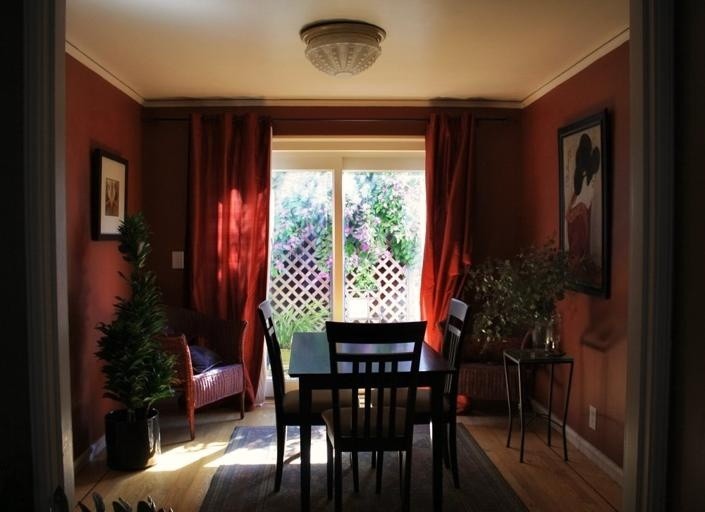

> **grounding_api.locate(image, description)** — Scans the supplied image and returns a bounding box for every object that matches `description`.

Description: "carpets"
[198,422,528,511]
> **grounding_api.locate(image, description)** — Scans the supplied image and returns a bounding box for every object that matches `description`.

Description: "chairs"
[257,299,360,501]
[458,333,531,413]
[322,320,427,510]
[371,298,472,496]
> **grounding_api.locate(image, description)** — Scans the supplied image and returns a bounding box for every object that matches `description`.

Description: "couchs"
[147,302,248,441]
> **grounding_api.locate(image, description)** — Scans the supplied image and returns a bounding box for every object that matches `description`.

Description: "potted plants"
[104,212,180,472]
[469,240,588,357]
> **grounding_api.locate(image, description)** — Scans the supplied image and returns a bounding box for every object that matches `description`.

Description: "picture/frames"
[558,108,612,300]
[91,146,129,241]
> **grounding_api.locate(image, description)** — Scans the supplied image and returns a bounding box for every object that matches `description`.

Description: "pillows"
[164,330,223,375]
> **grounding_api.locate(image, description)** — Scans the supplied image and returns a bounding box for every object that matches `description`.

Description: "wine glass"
[527,327,542,354]
[546,324,562,352]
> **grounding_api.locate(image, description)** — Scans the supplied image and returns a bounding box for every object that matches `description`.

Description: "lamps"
[300,21,387,78]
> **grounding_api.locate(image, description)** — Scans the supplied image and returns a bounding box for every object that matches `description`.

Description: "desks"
[501,347,574,464]
